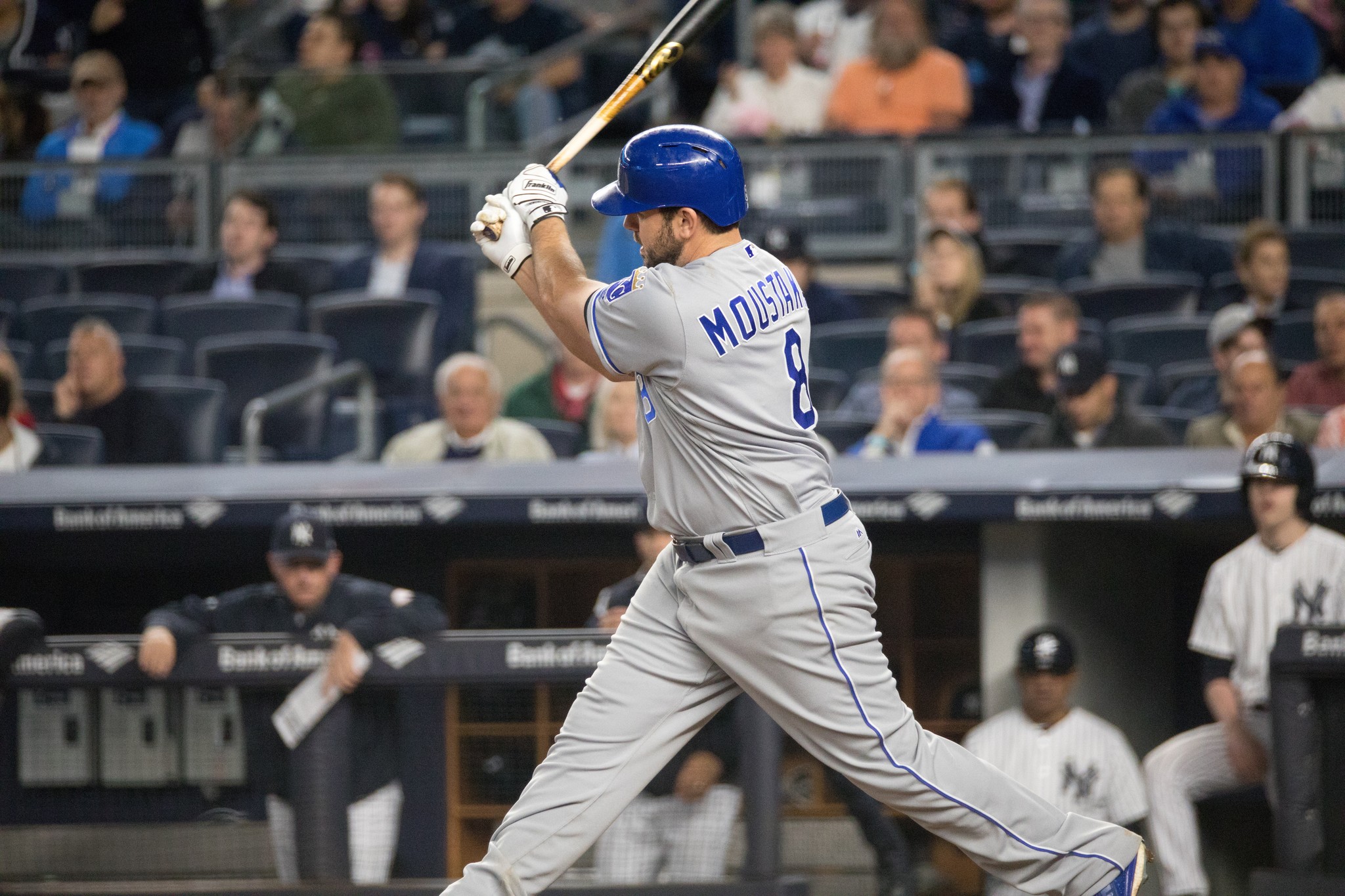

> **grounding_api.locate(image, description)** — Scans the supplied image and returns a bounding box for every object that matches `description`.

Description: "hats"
[74,50,124,95]
[1208,301,1272,352]
[269,511,335,564]
[1192,30,1236,63]
[1052,344,1108,396]
[1020,627,1077,677]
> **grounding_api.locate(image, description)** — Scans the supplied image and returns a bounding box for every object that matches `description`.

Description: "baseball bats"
[479,0,717,242]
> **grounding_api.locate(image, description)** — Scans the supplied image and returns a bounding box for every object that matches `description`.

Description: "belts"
[670,493,849,563]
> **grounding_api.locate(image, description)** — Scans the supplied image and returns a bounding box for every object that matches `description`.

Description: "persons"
[0,0,1345,896]
[434,124,1147,896]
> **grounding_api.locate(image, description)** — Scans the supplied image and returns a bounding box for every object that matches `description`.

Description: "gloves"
[507,162,568,230]
[471,193,533,278]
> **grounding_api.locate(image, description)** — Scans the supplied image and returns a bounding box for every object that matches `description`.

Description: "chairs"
[0,0,1345,462]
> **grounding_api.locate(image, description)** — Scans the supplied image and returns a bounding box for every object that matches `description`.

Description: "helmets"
[1237,432,1314,517]
[593,124,749,226]
